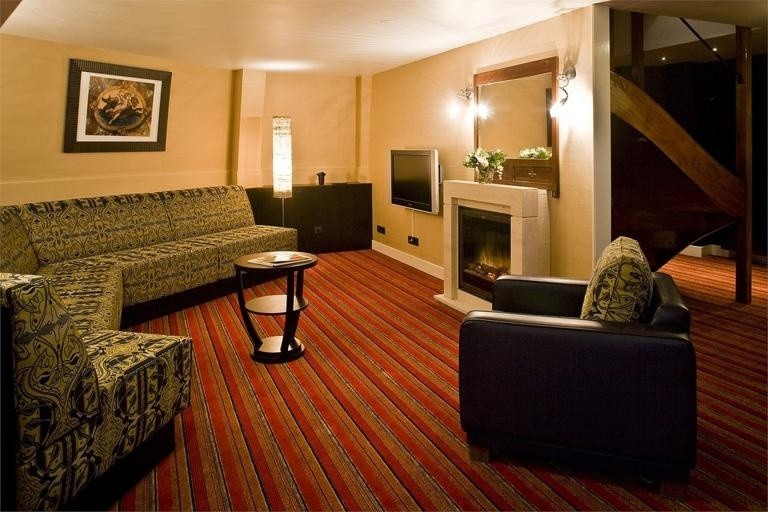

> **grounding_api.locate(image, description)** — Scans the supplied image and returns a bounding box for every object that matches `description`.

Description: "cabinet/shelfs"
[246,182,372,254]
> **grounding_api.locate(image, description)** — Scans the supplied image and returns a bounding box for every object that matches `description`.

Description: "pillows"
[580,235,654,322]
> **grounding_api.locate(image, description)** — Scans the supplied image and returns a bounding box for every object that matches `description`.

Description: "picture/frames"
[63,59,172,153]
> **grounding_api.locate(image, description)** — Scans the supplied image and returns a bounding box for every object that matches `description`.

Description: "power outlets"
[408,235,419,246]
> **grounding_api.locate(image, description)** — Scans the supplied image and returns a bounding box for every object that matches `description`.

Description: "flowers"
[518,146,552,159]
[462,147,508,181]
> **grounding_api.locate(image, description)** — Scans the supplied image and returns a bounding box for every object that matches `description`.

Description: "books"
[248,253,312,267]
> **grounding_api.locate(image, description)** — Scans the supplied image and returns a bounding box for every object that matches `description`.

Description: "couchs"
[459,271,697,492]
[0,185,298,511]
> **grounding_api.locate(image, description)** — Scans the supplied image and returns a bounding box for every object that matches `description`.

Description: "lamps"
[456,85,476,102]
[272,116,293,227]
[550,65,576,118]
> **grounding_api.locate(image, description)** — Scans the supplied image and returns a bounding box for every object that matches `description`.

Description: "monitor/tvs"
[390,149,442,215]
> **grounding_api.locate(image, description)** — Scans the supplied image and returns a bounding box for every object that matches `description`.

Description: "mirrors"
[472,56,559,198]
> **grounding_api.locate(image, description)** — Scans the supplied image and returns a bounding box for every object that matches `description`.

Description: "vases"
[478,169,494,183]
